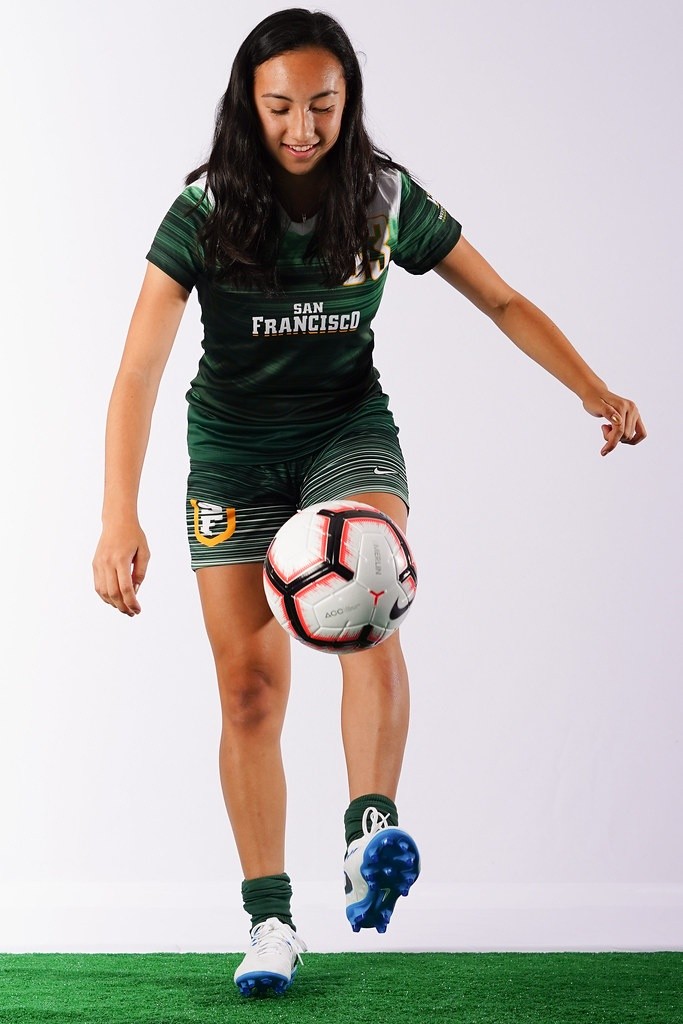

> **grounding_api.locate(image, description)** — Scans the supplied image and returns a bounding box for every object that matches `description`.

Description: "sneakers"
[234,918,307,997]
[340,807,421,935]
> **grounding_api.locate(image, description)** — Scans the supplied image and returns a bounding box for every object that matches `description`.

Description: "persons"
[93,8,647,995]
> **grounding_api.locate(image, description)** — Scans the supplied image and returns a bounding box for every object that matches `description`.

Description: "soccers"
[262,498,420,655]
